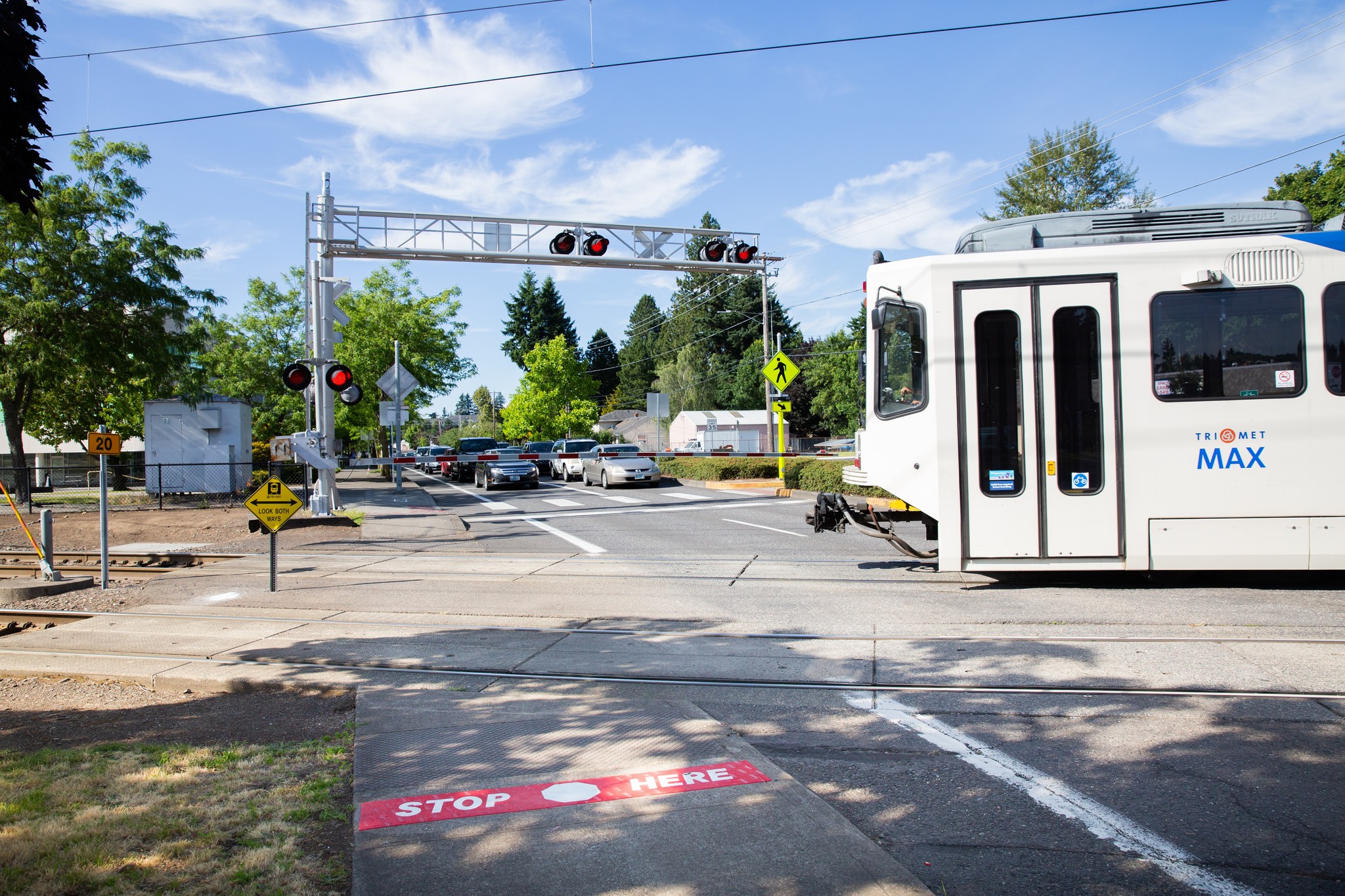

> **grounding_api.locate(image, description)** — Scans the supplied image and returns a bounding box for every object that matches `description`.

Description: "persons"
[900,387,923,405]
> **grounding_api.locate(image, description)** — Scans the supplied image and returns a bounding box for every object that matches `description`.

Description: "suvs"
[550,438,600,482]
[413,446,427,469]
[496,442,511,449]
[450,437,499,483]
[424,446,453,474]
[521,440,555,473]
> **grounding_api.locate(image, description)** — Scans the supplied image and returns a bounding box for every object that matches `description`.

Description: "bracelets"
[914,389,916,395]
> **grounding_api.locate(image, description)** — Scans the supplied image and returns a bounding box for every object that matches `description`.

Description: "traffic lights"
[283,363,354,391]
[706,241,758,264]
[553,232,610,256]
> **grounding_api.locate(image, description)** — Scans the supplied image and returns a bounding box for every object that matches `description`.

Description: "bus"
[799,198,1345,583]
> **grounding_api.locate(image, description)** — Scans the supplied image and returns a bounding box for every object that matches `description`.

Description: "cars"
[392,452,416,458]
[507,446,525,451]
[440,448,455,477]
[420,447,429,471]
[473,448,540,489]
[582,443,661,490]
